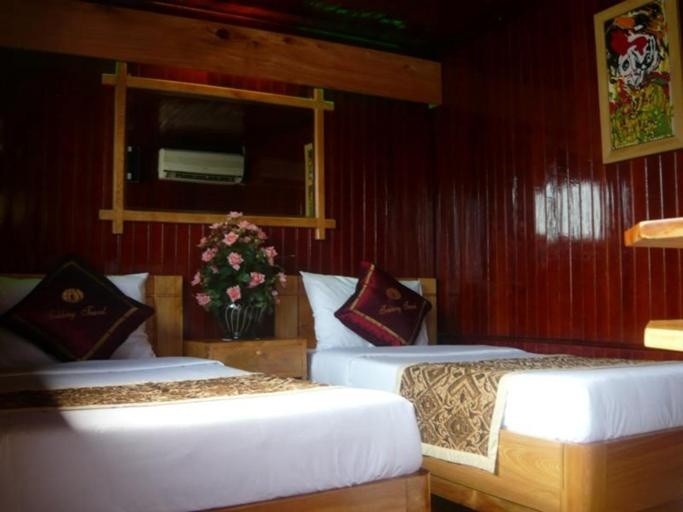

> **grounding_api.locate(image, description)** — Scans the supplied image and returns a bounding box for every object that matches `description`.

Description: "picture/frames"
[593,0,683,166]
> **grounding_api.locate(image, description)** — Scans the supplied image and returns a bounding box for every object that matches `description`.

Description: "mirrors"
[95,60,337,243]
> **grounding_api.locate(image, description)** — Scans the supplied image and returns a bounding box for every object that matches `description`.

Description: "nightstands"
[184,335,308,382]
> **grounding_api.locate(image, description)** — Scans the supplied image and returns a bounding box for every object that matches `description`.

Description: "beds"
[0,267,433,512]
[271,273,683,512]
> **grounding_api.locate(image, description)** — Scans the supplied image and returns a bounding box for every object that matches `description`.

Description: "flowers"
[189,208,288,310]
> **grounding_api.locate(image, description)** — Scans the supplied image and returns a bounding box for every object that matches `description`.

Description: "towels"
[0,372,330,416]
[399,353,667,475]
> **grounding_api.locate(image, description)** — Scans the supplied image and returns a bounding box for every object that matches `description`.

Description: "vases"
[215,297,263,340]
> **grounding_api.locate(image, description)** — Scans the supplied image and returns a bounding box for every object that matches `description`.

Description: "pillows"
[297,269,430,351]
[0,250,158,364]
[0,271,156,361]
[333,262,434,346]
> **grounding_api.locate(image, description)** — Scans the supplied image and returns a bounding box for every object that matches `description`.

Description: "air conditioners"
[157,148,246,186]
